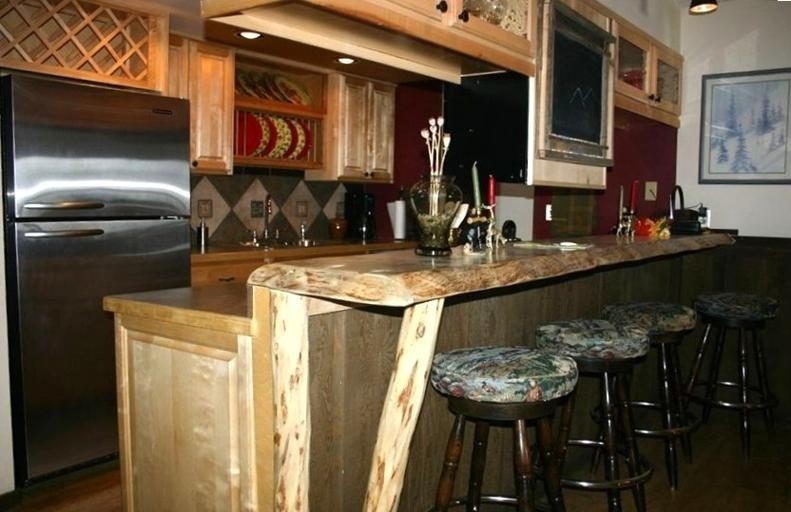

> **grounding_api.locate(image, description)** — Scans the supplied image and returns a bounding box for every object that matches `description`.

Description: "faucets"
[262,192,273,240]
[298,223,307,239]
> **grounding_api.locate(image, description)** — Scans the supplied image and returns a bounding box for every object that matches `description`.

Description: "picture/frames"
[696,68,791,185]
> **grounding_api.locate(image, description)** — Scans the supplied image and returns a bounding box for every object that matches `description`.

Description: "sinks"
[239,237,323,248]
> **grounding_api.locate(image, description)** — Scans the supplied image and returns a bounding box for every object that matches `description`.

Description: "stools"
[534,319,651,511]
[600,299,699,492]
[684,293,780,460]
[427,345,581,512]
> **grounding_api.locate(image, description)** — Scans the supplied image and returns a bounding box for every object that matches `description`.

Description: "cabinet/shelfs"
[0,1,171,98]
[303,71,398,184]
[233,48,328,170]
[616,15,684,118]
[190,255,267,286]
[201,0,539,87]
[168,30,234,176]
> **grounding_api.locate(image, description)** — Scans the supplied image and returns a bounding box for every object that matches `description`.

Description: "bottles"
[197,215,210,250]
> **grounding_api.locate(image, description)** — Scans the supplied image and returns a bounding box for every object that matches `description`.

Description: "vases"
[404,167,464,259]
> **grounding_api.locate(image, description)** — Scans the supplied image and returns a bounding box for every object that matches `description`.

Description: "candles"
[618,183,624,223]
[470,159,483,217]
[487,173,496,216]
[629,180,640,215]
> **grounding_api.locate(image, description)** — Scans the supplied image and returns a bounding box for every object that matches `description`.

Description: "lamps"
[686,0,718,15]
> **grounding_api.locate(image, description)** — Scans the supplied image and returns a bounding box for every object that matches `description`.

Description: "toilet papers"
[386,199,407,241]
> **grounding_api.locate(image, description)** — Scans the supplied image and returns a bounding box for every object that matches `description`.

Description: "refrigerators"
[0,71,193,491]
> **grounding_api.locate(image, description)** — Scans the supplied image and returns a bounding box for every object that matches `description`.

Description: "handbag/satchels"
[663,209,701,235]
